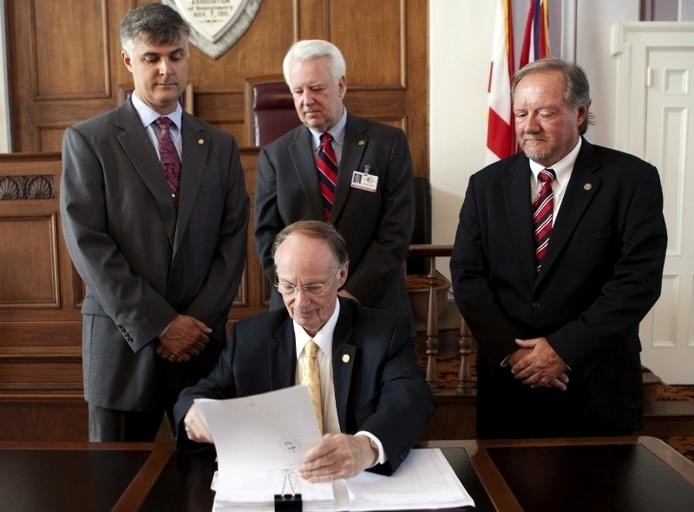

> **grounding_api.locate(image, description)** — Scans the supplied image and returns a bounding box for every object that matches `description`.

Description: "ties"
[303,341,323,436]
[315,133,339,225]
[154,117,181,207]
[531,168,556,273]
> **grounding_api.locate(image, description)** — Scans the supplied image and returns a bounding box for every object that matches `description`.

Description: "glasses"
[274,265,342,297]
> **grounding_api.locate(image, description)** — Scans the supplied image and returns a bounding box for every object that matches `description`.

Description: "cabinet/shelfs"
[8,2,428,151]
[0,155,263,391]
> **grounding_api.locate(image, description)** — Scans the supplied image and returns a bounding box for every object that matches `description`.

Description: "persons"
[449,58,668,438]
[60,4,250,445]
[171,220,436,486]
[253,39,415,338]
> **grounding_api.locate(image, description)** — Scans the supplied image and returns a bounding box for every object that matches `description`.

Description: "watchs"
[364,433,380,468]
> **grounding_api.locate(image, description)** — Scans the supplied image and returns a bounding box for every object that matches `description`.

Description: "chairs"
[238,71,313,149]
[401,179,452,322]
[115,82,194,127]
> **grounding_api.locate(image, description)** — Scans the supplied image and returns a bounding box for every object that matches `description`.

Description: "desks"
[3,436,694,511]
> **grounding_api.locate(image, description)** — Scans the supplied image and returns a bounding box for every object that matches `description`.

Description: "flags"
[518,0,552,72]
[486,0,514,161]
